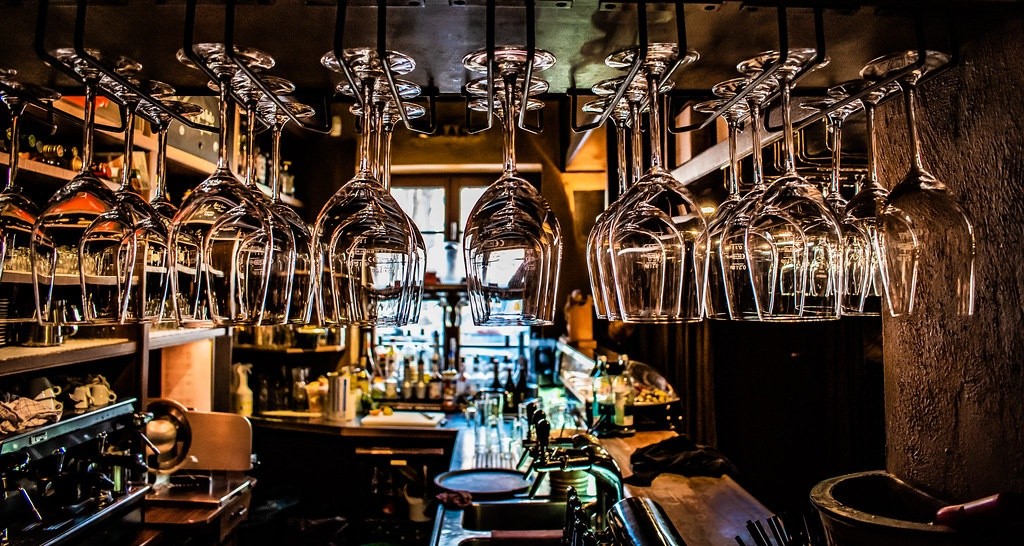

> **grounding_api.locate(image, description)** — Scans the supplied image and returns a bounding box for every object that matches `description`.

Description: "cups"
[92,385,117,405]
[32,377,61,399]
[473,392,524,460]
[74,386,94,409]
[39,397,64,423]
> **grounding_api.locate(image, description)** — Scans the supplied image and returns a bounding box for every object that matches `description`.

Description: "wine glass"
[0,41,976,330]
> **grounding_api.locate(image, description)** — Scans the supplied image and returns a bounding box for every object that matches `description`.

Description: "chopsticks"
[735,506,821,546]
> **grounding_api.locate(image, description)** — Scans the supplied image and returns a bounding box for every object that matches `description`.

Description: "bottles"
[275,365,291,408]
[593,356,614,438]
[293,369,307,407]
[359,353,531,412]
[612,354,636,434]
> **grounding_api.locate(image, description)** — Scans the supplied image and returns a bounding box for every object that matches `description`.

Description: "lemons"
[369,407,393,415]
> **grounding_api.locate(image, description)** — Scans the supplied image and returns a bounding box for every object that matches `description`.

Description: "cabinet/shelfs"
[0,314,149,546]
[0,96,306,376]
